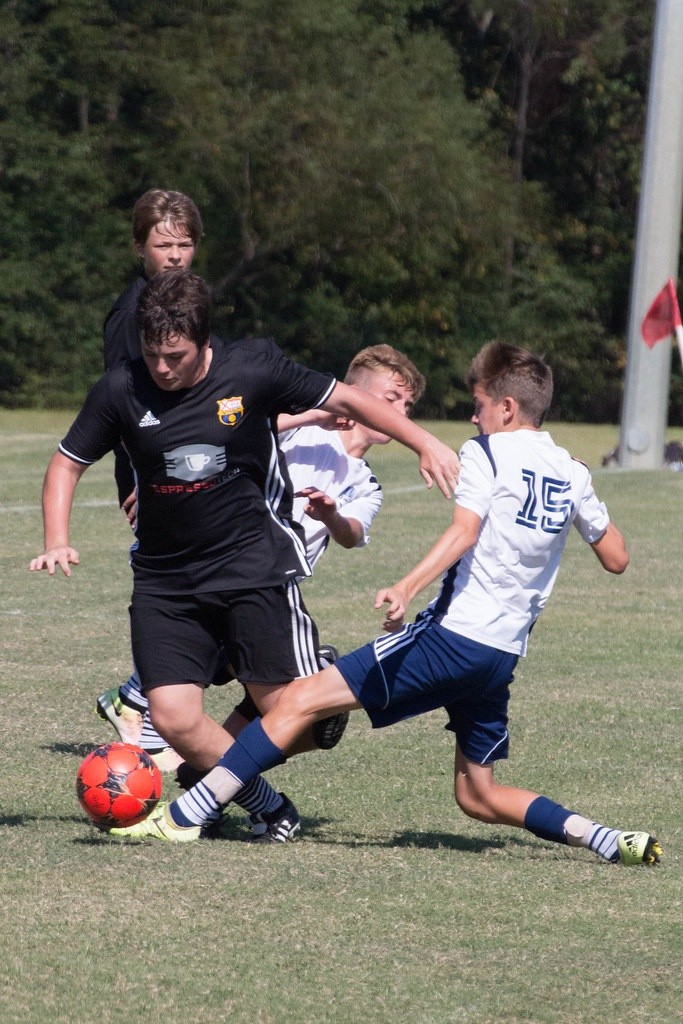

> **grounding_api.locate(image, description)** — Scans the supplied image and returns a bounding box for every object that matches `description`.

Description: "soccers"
[77,741,162,829]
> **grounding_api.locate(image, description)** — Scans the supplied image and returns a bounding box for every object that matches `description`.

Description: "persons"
[174,345,429,836]
[110,342,662,869]
[31,269,459,843]
[95,189,229,757]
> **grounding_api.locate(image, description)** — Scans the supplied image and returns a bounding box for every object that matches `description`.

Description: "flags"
[641,277,681,351]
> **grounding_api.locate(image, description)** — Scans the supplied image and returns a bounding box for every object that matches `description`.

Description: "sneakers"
[109,802,202,843]
[93,686,164,755]
[245,792,301,843]
[617,832,662,866]
[311,644,349,750]
